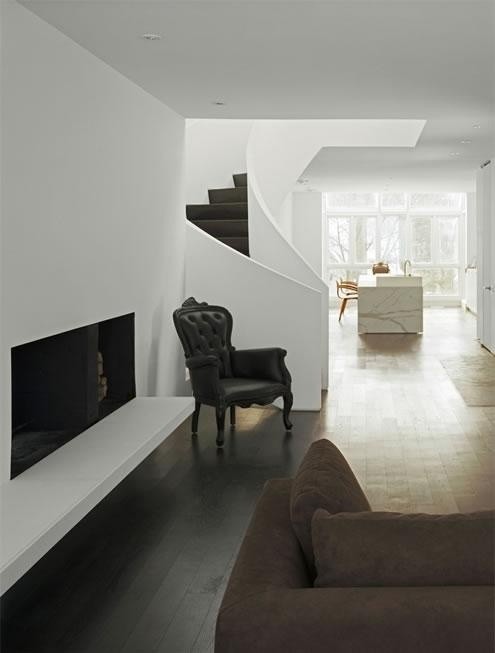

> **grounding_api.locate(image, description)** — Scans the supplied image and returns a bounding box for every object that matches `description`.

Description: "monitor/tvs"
[11,311,136,481]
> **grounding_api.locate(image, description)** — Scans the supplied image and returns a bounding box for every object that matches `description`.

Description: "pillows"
[288,438,373,576]
[305,504,494,587]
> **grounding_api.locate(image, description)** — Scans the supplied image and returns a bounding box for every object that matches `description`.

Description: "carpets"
[440,345,495,408]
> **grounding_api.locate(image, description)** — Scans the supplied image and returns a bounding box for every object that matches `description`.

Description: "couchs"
[211,437,494,651]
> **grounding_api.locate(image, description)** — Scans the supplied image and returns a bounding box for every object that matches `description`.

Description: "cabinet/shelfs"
[475,155,495,356]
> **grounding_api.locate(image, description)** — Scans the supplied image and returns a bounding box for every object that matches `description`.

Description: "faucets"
[404,259,411,276]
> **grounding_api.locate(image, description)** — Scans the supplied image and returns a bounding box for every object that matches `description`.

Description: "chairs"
[171,294,297,448]
[334,280,358,319]
[340,276,358,311]
